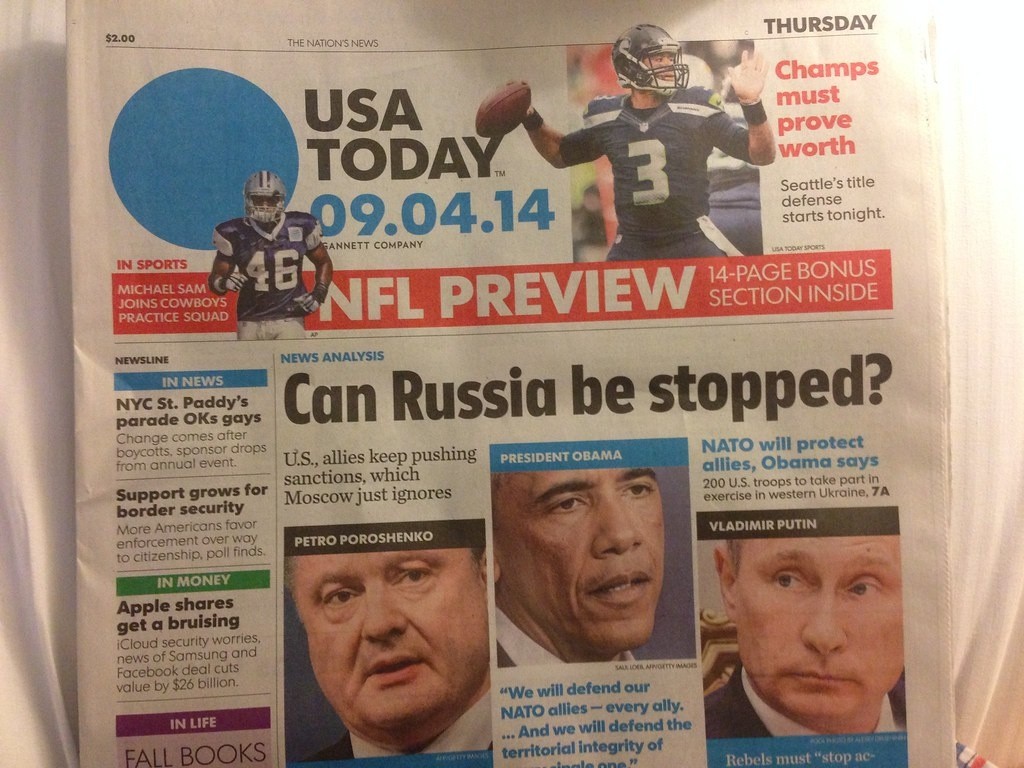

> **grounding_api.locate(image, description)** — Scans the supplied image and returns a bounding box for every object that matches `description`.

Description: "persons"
[504,24,779,262]
[285,547,492,768]
[208,173,334,340]
[705,536,906,738]
[491,468,665,666]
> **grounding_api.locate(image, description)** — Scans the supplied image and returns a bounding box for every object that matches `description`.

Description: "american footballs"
[475,82,531,138]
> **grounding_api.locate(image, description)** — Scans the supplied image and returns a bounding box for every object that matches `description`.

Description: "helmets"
[243,171,285,223]
[612,24,689,97]
[676,54,715,90]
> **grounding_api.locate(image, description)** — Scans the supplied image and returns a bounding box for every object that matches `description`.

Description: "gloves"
[216,274,248,293]
[293,283,328,316]
